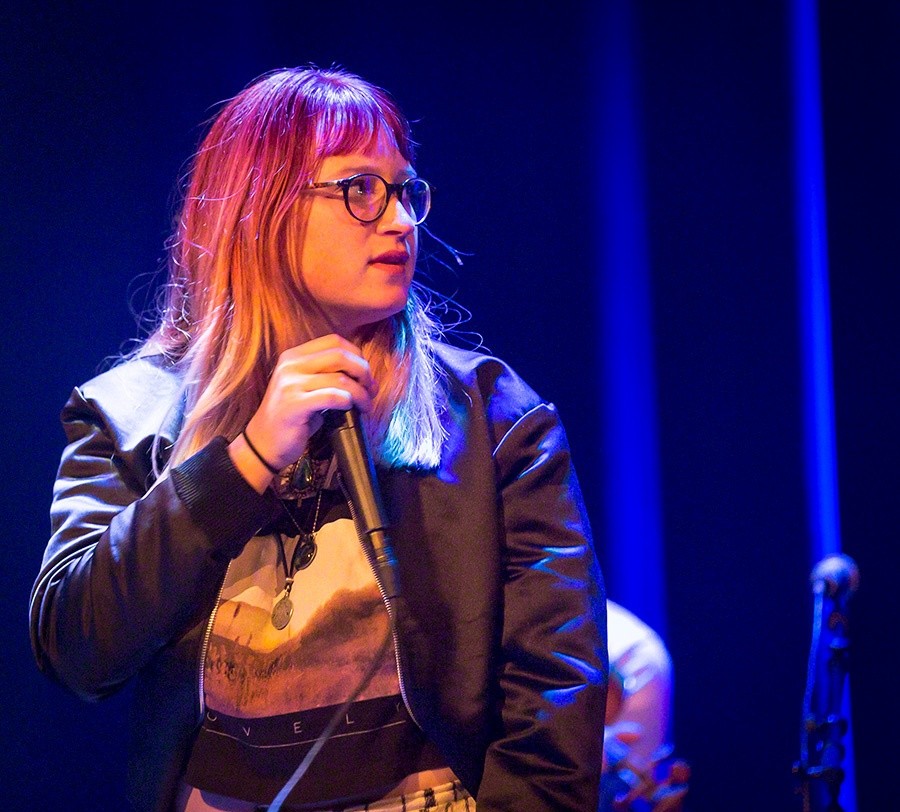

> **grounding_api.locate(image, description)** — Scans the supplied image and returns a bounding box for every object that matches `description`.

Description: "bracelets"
[242,424,283,481]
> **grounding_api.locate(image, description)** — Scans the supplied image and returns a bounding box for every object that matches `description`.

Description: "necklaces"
[260,426,336,630]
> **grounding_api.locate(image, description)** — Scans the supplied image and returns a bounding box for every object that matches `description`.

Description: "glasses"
[301,172,431,226]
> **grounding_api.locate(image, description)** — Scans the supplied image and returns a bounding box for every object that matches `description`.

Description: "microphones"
[315,404,403,599]
[811,554,861,597]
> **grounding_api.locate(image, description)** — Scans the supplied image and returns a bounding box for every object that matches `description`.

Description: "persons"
[596,602,683,812]
[26,65,610,812]
